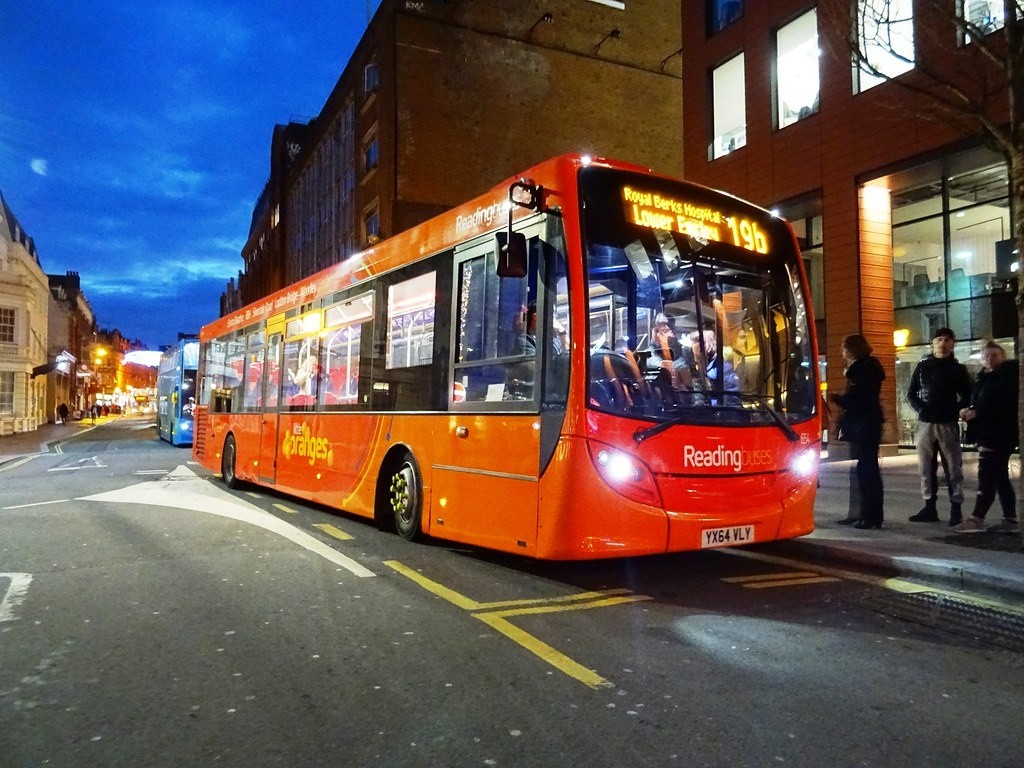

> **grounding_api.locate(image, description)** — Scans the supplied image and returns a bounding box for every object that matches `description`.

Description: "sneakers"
[987,518,1021,533]
[949,517,986,532]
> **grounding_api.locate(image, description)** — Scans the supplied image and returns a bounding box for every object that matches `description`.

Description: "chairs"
[216,357,359,406]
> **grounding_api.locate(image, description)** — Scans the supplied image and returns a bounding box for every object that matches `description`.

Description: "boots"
[950,503,962,526]
[908,499,939,522]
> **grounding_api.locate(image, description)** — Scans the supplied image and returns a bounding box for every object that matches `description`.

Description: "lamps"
[528,13,555,32]
[595,28,620,55]
[661,47,688,71]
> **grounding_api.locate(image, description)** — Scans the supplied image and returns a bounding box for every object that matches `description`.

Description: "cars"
[124,403,157,418]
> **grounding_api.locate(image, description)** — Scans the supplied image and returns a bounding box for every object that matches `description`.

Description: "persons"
[90,404,110,424]
[828,334,886,529]
[641,313,742,409]
[510,299,570,398]
[223,364,358,406]
[907,328,975,526]
[182,397,196,418]
[948,341,1021,533]
[59,403,68,424]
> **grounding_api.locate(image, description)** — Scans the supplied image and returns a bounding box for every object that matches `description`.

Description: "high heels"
[839,518,857,524]
[853,517,882,529]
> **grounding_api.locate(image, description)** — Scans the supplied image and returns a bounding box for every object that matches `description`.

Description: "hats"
[934,327,955,340]
[653,313,675,331]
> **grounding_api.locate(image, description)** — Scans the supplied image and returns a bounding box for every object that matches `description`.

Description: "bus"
[156,338,264,446]
[192,152,825,564]
